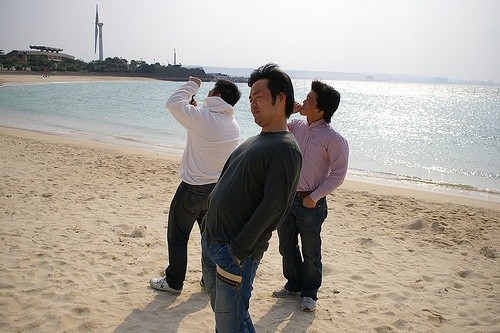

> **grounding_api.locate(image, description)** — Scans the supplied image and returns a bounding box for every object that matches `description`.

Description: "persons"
[200,63,303,333]
[272,80,349,312]
[149,76,242,295]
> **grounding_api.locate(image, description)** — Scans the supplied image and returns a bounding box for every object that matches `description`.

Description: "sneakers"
[149,276,182,293]
[272,286,301,297]
[300,296,316,311]
